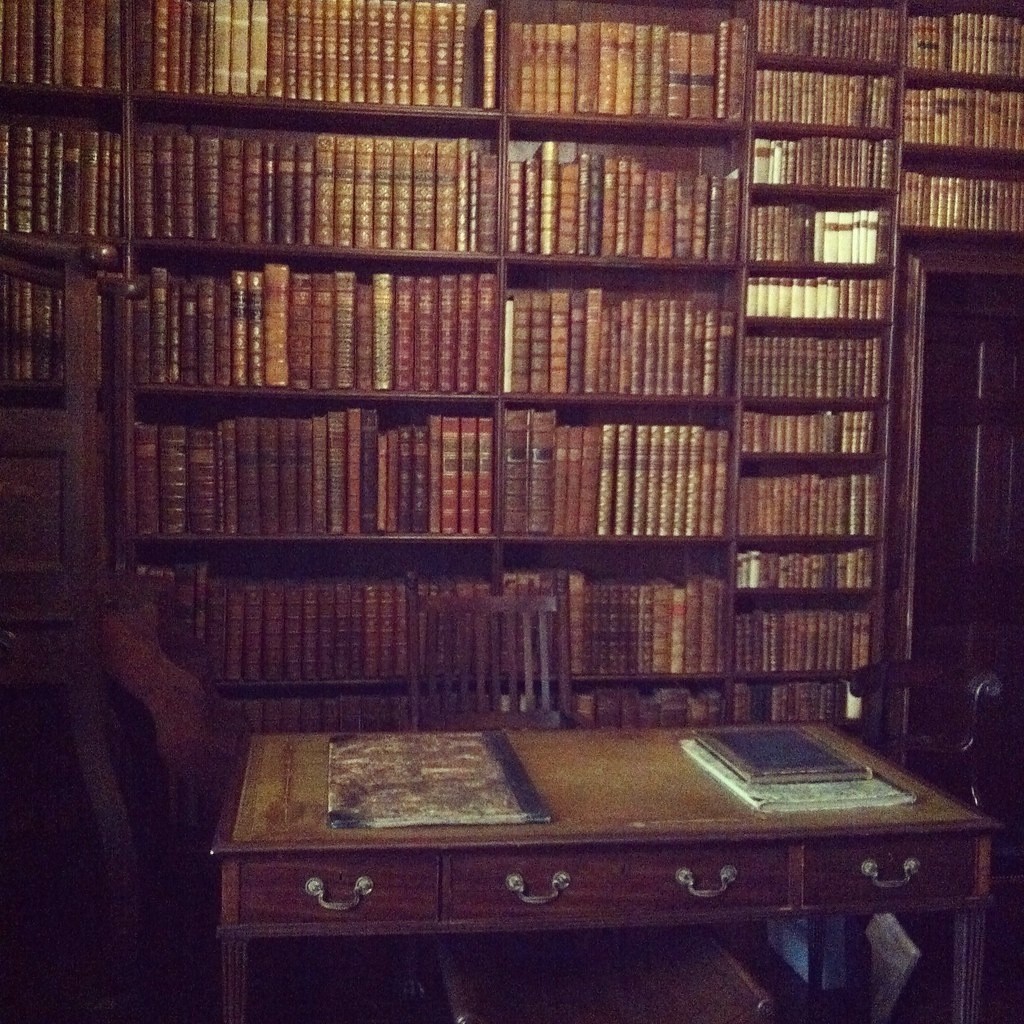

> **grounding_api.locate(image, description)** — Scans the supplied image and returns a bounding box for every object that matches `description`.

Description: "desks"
[211,721,1005,1024]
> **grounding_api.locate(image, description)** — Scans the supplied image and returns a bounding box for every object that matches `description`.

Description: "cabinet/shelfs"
[0,0,1022,855]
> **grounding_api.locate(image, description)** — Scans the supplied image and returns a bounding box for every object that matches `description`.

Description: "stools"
[443,925,779,1024]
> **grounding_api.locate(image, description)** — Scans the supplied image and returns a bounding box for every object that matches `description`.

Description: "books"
[324,733,546,830]
[696,727,874,787]
[1,0,1023,731]
[679,731,921,816]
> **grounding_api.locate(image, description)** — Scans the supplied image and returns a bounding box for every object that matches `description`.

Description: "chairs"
[399,565,570,743]
[804,662,1004,1024]
[75,557,407,1023]
